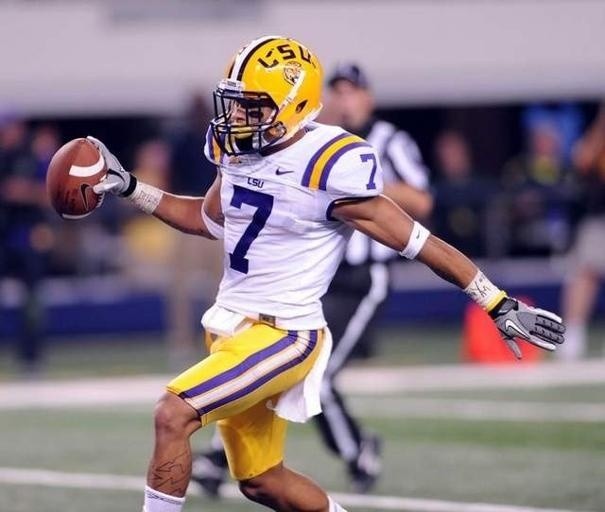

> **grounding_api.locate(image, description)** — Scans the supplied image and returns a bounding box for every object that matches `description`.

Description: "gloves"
[487,295,566,359]
[86,135,137,198]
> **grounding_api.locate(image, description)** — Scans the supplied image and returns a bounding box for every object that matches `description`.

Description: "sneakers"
[190,452,229,494]
[349,437,383,494]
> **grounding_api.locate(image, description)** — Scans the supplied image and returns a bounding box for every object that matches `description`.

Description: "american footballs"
[47,138,108,222]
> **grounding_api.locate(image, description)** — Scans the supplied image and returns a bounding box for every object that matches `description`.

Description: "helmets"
[210,35,323,157]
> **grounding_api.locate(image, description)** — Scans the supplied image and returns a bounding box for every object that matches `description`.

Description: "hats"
[327,65,366,89]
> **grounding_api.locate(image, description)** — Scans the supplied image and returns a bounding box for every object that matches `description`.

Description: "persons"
[423,96,605,362]
[2,91,224,376]
[84,34,566,512]
[189,59,436,502]
